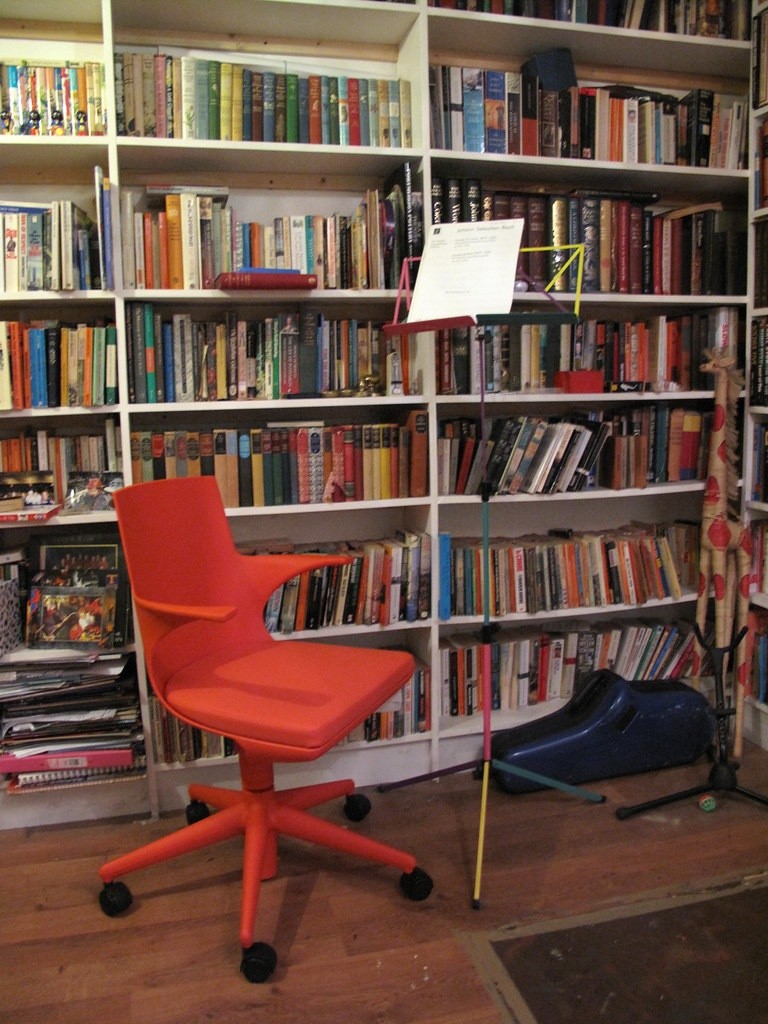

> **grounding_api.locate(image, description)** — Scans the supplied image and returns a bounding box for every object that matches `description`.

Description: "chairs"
[98,476,437,984]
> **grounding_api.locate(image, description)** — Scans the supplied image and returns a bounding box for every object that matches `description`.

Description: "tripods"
[378,246,607,913]
[617,624,768,821]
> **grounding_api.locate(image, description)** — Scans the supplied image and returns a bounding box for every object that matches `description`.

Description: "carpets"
[456,866,768,1024]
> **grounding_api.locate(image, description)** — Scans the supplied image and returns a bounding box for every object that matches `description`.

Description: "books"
[0,0,768,797]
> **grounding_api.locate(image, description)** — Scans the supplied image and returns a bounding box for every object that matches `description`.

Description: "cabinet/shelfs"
[729,0,768,750]
[0,0,751,832]
